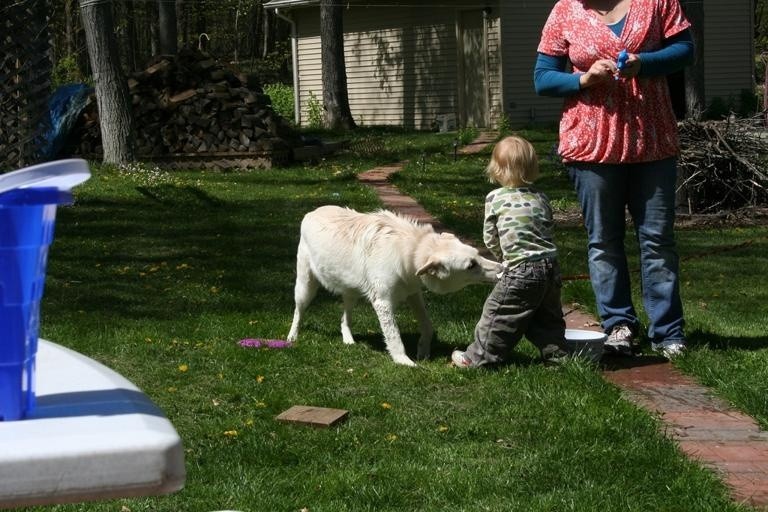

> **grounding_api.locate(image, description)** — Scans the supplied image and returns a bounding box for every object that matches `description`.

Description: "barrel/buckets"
[0,185,76,421]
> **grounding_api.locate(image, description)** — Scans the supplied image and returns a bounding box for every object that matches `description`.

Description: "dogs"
[286,204,504,368]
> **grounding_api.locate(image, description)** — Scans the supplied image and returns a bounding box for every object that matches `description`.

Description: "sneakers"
[451,349,472,369]
[542,343,569,371]
[656,344,689,363]
[602,323,633,357]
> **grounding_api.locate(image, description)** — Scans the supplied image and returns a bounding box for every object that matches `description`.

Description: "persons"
[533,0,701,364]
[450,136,571,369]
